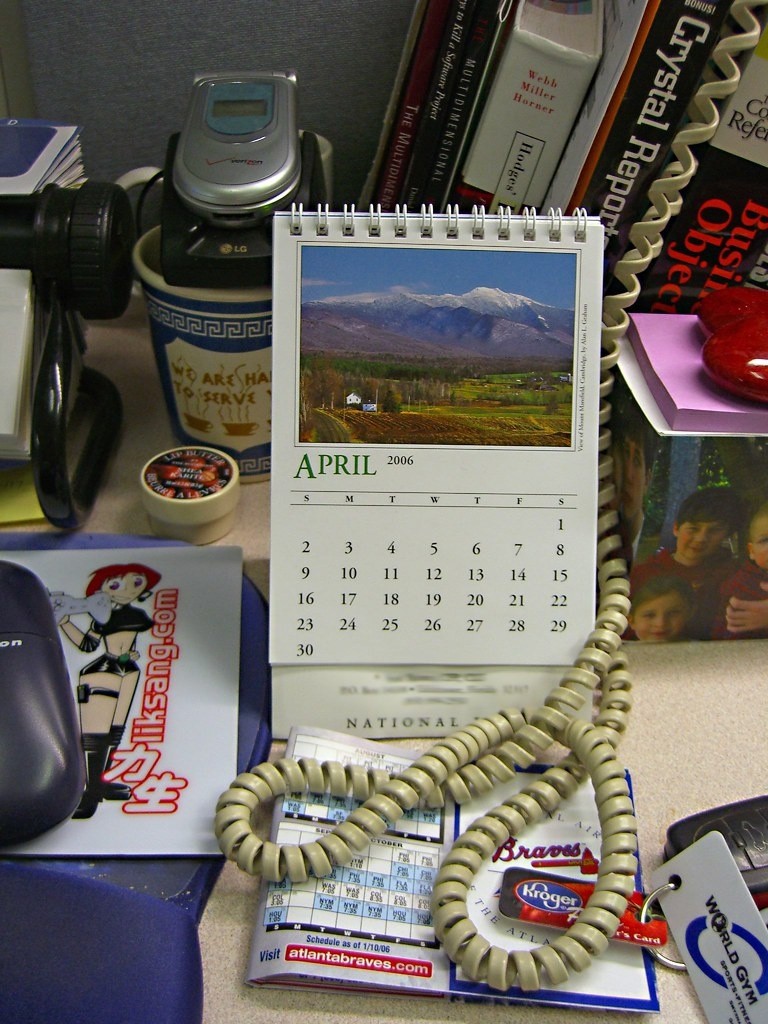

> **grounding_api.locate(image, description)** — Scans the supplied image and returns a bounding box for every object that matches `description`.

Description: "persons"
[615,398,768,643]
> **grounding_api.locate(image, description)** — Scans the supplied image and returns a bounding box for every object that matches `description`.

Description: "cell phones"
[173,69,301,228]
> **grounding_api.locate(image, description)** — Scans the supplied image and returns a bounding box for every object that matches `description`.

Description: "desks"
[0,276,768,1024]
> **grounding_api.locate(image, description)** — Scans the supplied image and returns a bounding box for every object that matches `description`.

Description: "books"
[375,2,768,321]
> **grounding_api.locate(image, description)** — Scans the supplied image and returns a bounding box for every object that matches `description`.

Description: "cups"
[136,225,272,483]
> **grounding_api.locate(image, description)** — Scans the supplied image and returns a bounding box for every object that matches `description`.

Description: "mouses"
[0,559,87,848]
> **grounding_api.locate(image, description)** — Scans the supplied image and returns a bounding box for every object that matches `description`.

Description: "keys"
[662,796,768,898]
